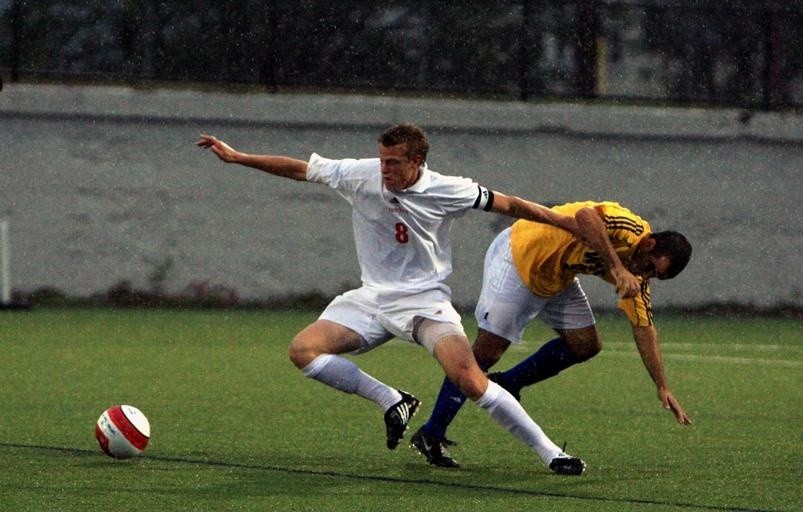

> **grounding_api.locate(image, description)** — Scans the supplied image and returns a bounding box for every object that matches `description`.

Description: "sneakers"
[548,454,586,475]
[410,425,462,468]
[487,372,520,401]
[384,389,422,450]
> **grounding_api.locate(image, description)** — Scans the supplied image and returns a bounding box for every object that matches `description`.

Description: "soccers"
[96,405,151,459]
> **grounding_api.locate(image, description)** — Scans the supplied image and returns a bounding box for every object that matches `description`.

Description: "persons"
[0,1,802,112]
[408,200,692,470]
[192,120,589,478]
